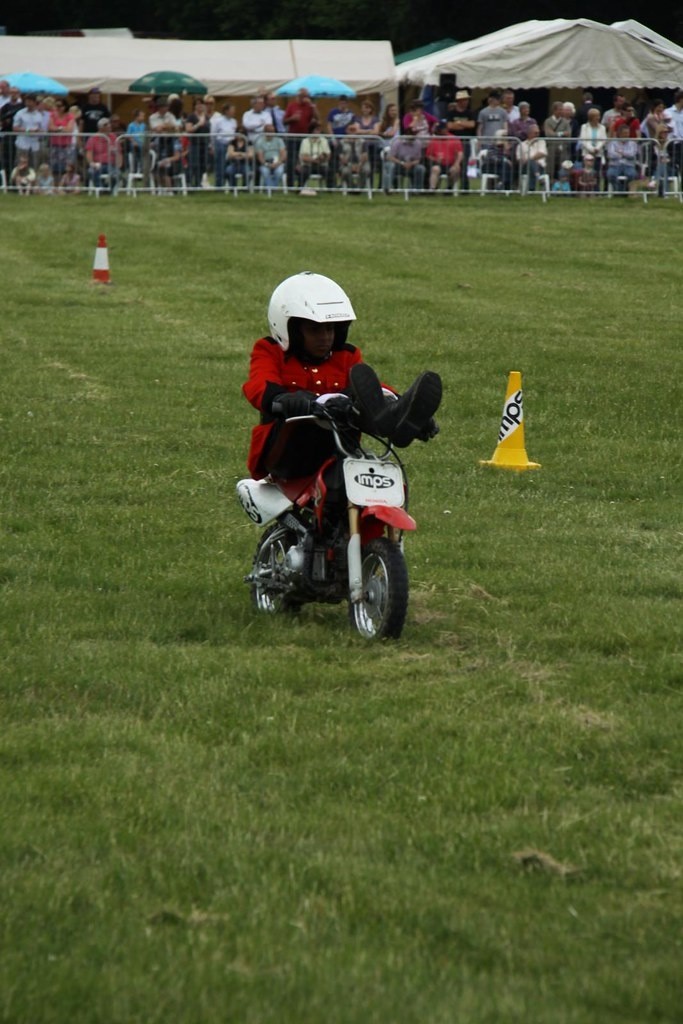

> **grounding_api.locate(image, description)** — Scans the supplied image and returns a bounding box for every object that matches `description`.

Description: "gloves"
[417,418,439,442]
[314,393,350,422]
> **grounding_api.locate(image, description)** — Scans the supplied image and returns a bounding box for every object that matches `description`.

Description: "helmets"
[268,271,357,351]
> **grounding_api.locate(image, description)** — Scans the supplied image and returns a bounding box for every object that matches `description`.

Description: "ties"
[270,108,278,132]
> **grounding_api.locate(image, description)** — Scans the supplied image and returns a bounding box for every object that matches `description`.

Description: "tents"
[0,18,683,118]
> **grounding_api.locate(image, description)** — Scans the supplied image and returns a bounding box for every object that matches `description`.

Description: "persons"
[0,81,683,197]
[242,270,442,481]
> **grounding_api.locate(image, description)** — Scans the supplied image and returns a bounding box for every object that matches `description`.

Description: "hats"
[560,169,568,176]
[655,123,669,138]
[25,92,36,100]
[456,91,470,99]
[585,154,593,159]
[563,159,574,169]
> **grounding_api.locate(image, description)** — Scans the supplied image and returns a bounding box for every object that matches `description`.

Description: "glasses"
[236,130,245,133]
[56,104,64,108]
[626,108,634,112]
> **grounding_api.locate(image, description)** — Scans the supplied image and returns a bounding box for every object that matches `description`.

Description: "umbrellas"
[128,71,208,95]
[273,73,356,104]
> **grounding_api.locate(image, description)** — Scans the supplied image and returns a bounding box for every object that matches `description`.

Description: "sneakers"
[350,364,443,447]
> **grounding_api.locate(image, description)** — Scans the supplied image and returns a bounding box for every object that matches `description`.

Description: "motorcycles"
[243,402,441,641]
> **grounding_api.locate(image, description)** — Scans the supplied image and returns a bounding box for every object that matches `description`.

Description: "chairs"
[0,149,683,201]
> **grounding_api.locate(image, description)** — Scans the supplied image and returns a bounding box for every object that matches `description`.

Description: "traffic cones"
[93,235,110,284]
[478,371,540,469]
